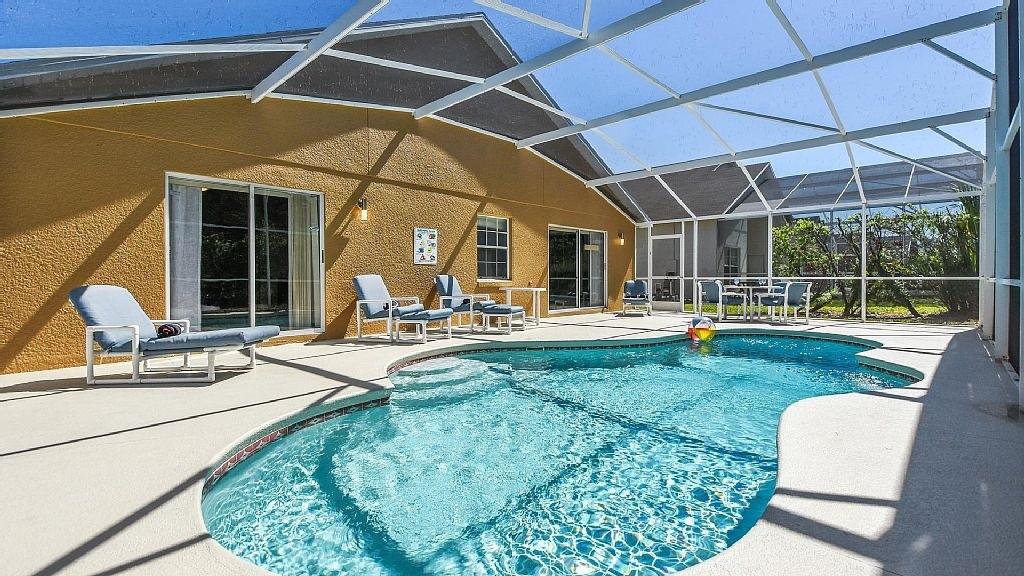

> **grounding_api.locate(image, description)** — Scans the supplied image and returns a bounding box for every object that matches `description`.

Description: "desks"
[724,285,783,321]
[499,286,546,326]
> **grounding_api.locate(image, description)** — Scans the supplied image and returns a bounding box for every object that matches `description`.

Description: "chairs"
[698,280,812,325]
[353,273,454,344]
[434,274,527,334]
[66,284,280,384]
[622,280,652,316]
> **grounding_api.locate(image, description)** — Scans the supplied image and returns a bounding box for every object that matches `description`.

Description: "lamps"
[357,197,368,220]
[617,232,624,245]
